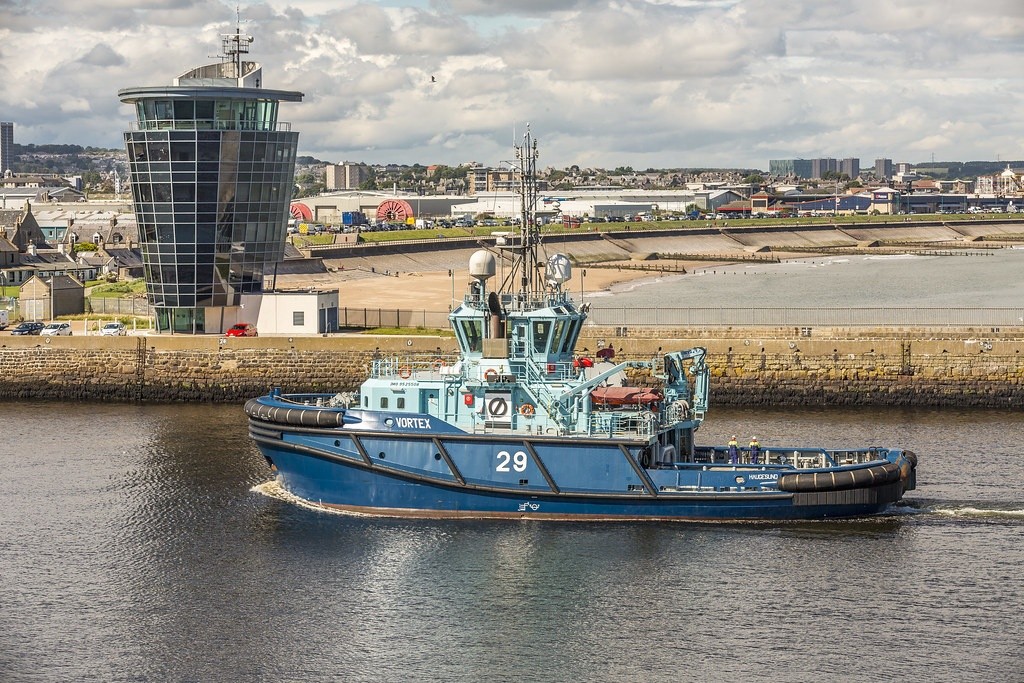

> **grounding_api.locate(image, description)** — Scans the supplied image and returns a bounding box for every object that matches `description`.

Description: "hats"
[732,435,736,439]
[753,436,756,440]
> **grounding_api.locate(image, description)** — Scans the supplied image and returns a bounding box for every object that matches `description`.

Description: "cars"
[10,321,46,335]
[100,323,128,336]
[284,205,1024,236]
[39,322,73,336]
[225,323,258,336]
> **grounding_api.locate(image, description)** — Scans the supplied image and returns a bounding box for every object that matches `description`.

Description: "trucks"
[0,309,9,330]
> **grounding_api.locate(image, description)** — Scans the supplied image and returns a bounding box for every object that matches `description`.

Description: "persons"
[727,435,742,464]
[748,436,761,465]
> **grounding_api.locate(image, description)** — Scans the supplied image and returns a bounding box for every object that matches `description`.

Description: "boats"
[244,120,920,522]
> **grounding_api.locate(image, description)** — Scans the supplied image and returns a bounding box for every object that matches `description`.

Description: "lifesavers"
[399,365,411,379]
[521,403,534,415]
[434,359,445,370]
[484,368,497,380]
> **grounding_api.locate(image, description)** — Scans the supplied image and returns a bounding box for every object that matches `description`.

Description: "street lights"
[116,255,120,281]
[28,275,37,322]
[45,276,54,323]
